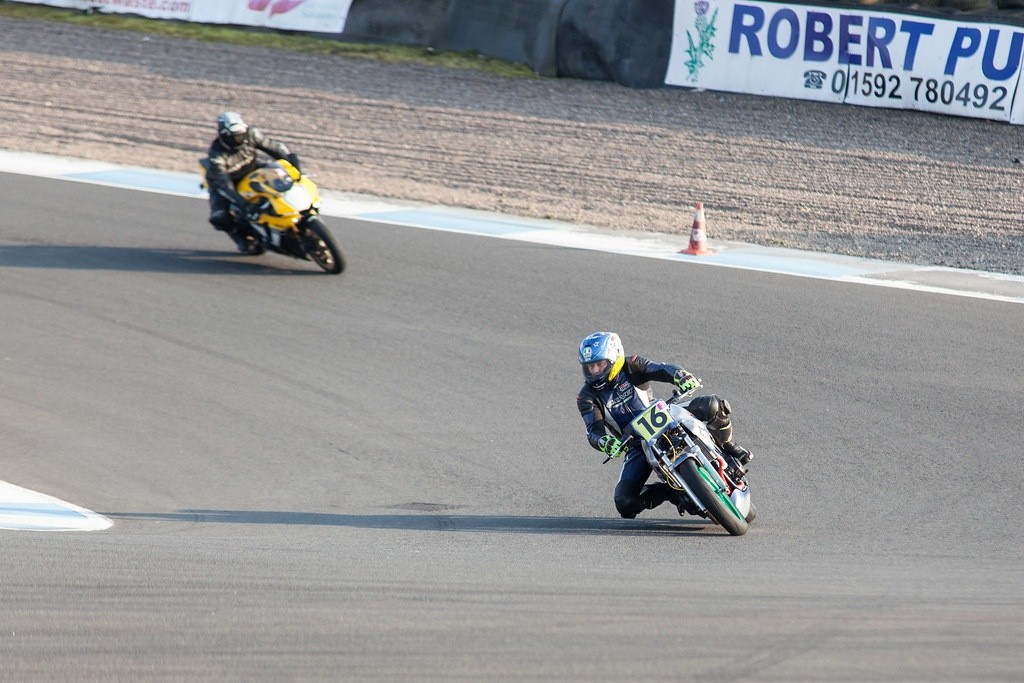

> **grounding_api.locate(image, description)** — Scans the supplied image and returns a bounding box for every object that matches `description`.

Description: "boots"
[706,420,747,461]
[644,483,686,509]
[229,226,249,251]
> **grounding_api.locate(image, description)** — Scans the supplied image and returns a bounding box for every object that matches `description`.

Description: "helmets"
[217,111,248,149]
[578,331,626,389]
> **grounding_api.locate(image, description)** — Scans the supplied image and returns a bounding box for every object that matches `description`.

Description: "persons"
[576,332,754,519]
[206,112,301,252]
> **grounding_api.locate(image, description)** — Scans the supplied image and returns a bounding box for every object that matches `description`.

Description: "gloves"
[598,435,629,458]
[674,370,699,393]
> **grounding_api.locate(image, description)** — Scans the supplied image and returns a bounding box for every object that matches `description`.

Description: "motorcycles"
[198,157,348,276]
[602,379,758,536]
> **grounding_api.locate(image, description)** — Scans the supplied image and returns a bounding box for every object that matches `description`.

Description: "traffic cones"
[676,199,719,258]
